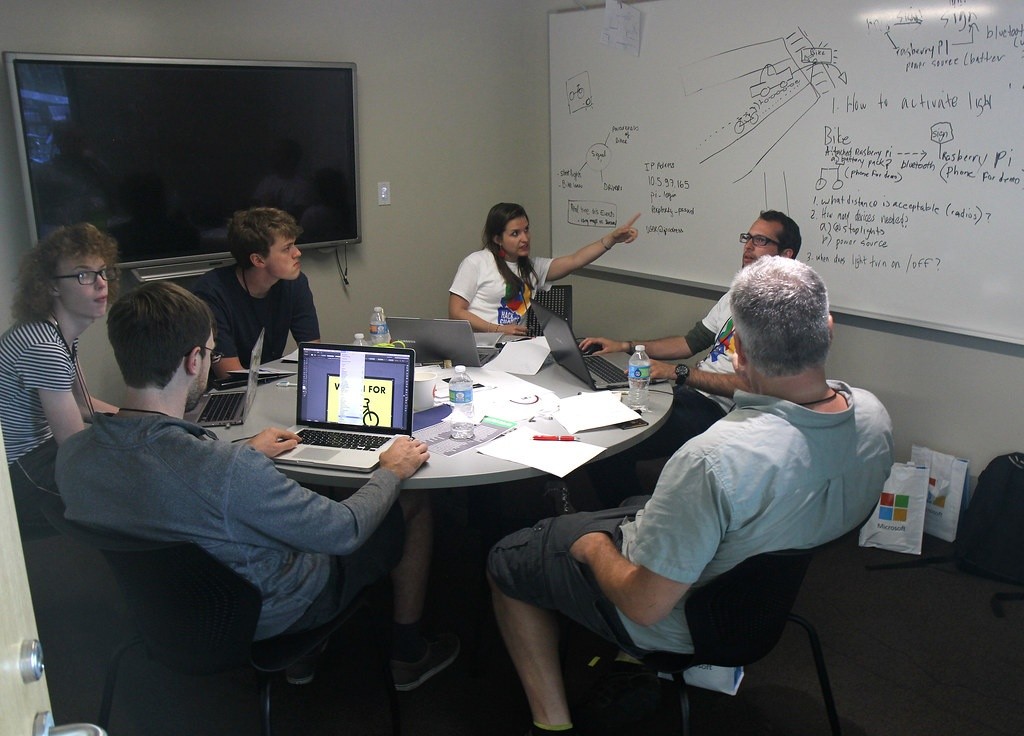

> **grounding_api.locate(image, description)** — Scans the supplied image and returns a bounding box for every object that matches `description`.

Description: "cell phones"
[617,419,649,429]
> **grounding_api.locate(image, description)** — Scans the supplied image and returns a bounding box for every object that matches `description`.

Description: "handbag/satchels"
[909,446,970,544]
[859,462,930,555]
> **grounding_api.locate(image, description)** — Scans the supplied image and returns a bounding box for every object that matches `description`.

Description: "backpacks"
[954,452,1024,586]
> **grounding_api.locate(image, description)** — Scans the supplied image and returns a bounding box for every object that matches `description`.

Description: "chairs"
[528,283,571,338]
[556,495,880,736]
[41,501,394,736]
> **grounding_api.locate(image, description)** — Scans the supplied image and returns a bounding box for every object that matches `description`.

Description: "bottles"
[368,306,390,345]
[449,364,475,442]
[626,344,650,412]
[353,332,367,345]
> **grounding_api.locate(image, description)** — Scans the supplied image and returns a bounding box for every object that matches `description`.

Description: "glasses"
[184,346,223,363]
[741,233,778,247]
[50,268,117,285]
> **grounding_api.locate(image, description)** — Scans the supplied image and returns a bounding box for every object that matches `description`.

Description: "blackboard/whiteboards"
[548,0,1024,346]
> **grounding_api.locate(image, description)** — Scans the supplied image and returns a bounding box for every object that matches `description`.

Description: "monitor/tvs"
[1,52,361,271]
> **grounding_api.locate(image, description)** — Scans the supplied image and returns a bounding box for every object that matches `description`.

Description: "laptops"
[182,328,265,428]
[385,317,498,368]
[528,298,670,392]
[272,342,415,472]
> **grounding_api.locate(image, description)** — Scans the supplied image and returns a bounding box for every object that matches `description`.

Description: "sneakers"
[389,633,460,692]
[286,639,328,684]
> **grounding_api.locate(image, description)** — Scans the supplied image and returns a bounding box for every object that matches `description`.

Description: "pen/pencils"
[276,381,297,387]
[533,435,580,441]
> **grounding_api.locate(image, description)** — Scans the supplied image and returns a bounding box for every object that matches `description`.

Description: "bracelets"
[488,323,492,333]
[627,340,636,353]
[495,324,501,333]
[601,237,609,251]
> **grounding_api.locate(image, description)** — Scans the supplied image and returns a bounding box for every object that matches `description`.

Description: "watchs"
[675,364,689,385]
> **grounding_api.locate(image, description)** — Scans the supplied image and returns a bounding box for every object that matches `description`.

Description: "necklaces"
[796,388,836,406]
[51,311,95,418]
[242,267,251,295]
[119,407,174,418]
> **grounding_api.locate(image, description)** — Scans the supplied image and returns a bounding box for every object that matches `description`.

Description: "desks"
[202,335,674,556]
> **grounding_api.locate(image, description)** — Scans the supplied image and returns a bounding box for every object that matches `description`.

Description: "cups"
[413,372,438,412]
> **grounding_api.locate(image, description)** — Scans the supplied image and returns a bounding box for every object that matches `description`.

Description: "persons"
[52,280,461,693]
[0,222,121,514]
[448,202,648,338]
[183,208,322,379]
[486,254,895,736]
[576,210,801,399]
